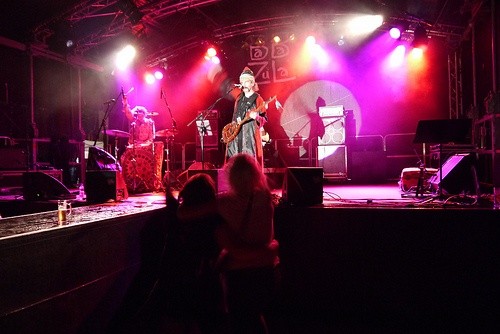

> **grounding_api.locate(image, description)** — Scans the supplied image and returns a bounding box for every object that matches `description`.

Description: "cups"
[493,187,500,209]
[57,199,72,224]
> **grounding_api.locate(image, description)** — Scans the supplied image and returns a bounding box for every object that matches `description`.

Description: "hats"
[178,174,216,204]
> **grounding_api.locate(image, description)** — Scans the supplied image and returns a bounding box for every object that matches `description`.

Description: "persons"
[225,68,268,179]
[125,106,155,148]
[148,155,280,334]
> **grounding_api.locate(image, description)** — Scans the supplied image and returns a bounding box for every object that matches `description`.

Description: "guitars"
[221,95,277,143]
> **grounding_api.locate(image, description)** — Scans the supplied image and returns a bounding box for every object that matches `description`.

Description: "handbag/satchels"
[157,286,210,323]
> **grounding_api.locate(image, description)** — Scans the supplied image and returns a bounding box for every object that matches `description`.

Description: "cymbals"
[155,129,179,137]
[101,130,131,137]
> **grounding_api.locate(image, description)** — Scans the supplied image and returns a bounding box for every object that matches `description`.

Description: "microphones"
[161,88,163,99]
[234,84,242,88]
[104,99,116,105]
[121,88,125,98]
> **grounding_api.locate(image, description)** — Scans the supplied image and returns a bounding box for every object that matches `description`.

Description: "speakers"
[430,154,477,199]
[281,118,348,207]
[86,170,117,203]
[194,119,221,167]
[22,172,71,201]
